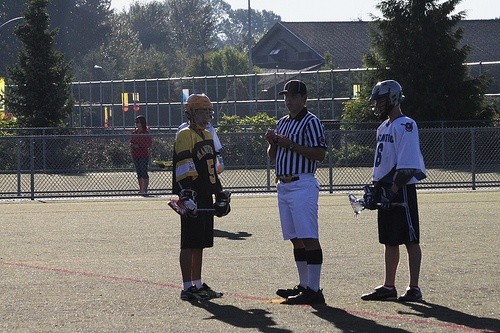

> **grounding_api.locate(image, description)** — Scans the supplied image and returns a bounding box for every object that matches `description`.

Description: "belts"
[275,174,314,183]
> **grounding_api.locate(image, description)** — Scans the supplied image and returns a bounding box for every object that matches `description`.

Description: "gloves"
[380,187,399,210]
[213,190,231,217]
[215,156,224,174]
[363,184,380,210]
[176,190,198,215]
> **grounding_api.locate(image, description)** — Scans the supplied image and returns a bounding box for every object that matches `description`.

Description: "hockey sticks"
[167,199,216,216]
[349,193,407,215]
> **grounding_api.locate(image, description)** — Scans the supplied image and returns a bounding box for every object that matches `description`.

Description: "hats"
[279,80,307,95]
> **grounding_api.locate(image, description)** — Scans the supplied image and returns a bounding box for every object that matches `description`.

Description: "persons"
[171,94,230,301]
[265,80,328,303]
[361,80,426,302]
[130,116,154,194]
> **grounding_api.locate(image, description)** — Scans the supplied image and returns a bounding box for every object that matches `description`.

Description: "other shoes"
[198,283,223,298]
[276,284,305,299]
[397,288,422,303]
[361,285,398,301]
[181,284,210,301]
[288,286,325,305]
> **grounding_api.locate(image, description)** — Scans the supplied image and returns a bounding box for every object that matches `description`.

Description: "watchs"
[289,141,294,151]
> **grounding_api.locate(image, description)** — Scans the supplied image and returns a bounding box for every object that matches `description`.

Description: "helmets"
[184,93,213,113]
[368,79,406,120]
